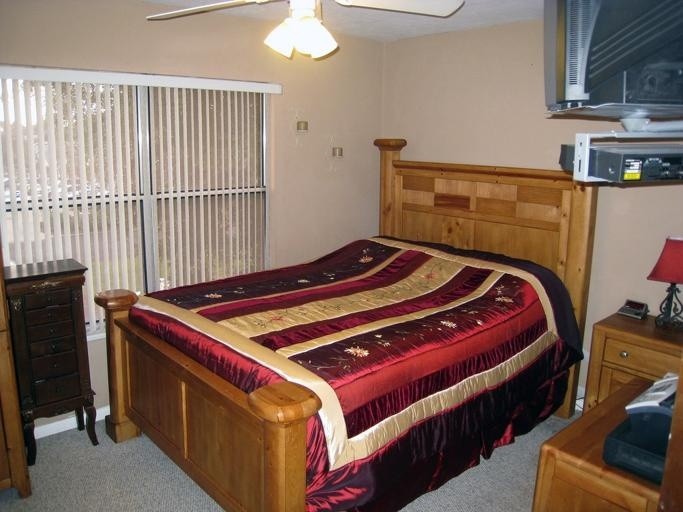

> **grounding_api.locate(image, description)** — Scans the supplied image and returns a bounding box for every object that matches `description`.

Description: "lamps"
[646,236,683,332]
[264,0,338,60]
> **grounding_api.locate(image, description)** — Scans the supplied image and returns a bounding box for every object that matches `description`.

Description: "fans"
[146,0,466,18]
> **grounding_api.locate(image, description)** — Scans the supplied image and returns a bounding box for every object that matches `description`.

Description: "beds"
[94,138,599,511]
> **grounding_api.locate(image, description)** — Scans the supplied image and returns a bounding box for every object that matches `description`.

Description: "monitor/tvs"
[555,0,682,108]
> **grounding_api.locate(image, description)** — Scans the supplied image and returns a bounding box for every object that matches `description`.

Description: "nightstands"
[581,312,683,414]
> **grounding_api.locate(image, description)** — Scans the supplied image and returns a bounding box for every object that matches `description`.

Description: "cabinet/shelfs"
[4,259,99,466]
[532,376,683,512]
